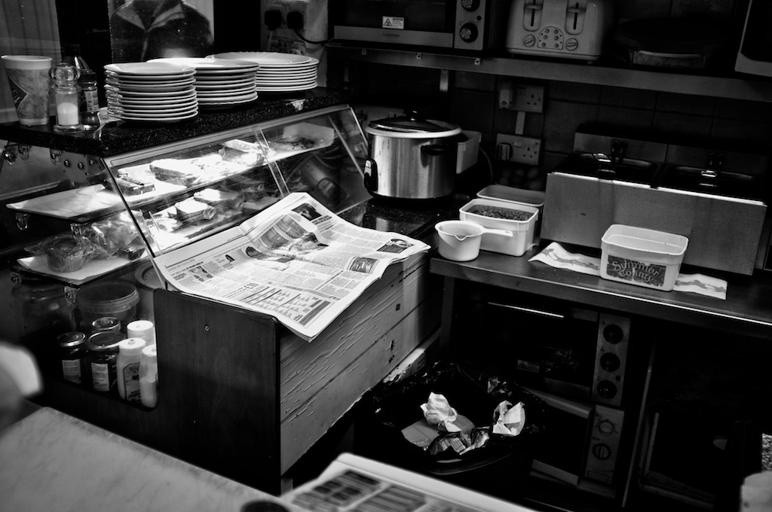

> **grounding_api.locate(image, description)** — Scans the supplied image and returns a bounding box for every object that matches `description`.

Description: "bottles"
[12,284,74,381]
[49,64,79,130]
[118,319,157,406]
[78,70,102,127]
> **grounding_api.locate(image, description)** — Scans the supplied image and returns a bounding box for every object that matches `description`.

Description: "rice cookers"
[359,110,463,210]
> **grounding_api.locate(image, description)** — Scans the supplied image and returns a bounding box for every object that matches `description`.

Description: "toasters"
[504,1,605,64]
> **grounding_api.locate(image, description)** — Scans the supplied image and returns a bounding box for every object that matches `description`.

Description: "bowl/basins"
[75,281,138,329]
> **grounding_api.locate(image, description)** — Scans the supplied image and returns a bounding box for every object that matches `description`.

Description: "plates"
[102,52,318,124]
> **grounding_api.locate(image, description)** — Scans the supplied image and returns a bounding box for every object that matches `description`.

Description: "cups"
[3,56,53,124]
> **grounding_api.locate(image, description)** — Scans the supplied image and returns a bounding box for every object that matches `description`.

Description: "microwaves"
[334,0,489,56]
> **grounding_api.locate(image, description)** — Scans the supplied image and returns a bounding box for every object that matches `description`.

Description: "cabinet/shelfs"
[0,84,369,475]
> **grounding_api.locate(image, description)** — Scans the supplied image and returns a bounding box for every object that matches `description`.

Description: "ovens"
[475,287,633,407]
[520,384,624,497]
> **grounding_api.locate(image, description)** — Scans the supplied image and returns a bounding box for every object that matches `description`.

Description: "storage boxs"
[594,224,691,291]
[475,182,547,212]
[456,198,538,259]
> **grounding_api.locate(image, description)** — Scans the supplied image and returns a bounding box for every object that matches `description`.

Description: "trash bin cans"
[355,375,540,503]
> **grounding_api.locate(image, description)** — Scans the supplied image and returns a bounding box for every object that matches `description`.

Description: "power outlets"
[494,131,540,168]
[499,85,545,113]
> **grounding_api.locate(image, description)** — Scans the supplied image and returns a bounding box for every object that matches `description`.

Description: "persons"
[299,205,321,221]
[246,246,292,263]
[385,240,412,249]
[110,0,212,64]
[225,255,235,262]
